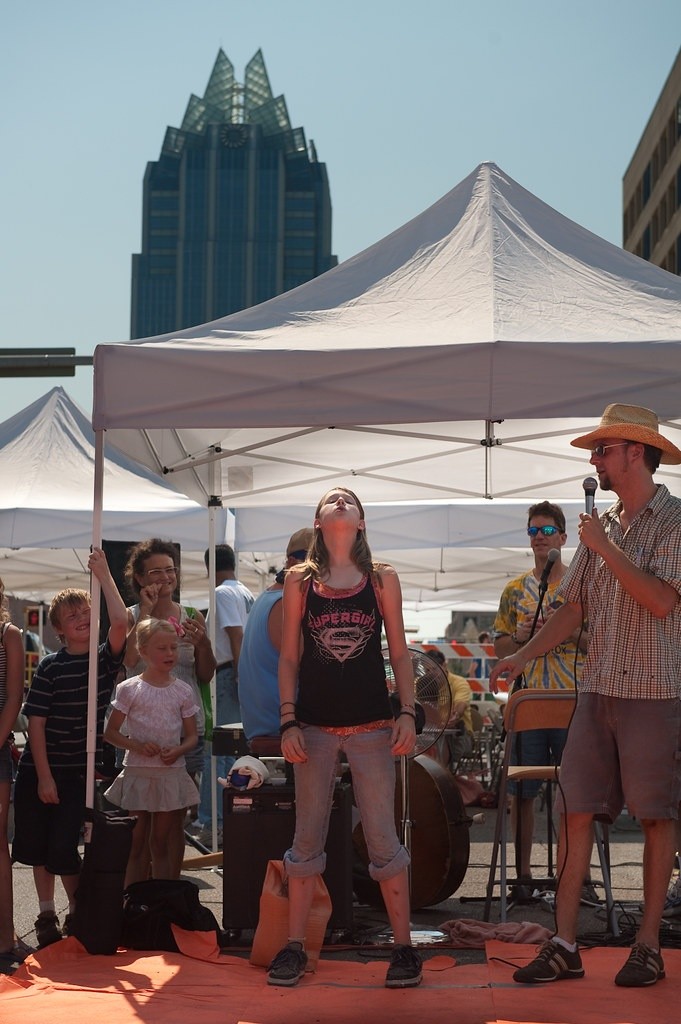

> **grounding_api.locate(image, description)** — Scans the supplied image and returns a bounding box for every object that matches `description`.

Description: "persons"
[0,577,24,954]
[490,403,681,987]
[420,649,475,774]
[103,528,315,913]
[478,631,490,643]
[12,547,129,948]
[267,486,425,988]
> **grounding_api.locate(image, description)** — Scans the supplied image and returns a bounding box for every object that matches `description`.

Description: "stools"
[482,688,627,938]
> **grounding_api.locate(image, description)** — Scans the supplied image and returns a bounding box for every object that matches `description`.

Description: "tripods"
[460,582,596,918]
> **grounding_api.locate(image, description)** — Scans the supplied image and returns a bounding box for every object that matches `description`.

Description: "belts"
[217,661,235,674]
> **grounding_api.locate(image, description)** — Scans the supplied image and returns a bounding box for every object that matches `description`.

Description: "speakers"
[223,783,353,946]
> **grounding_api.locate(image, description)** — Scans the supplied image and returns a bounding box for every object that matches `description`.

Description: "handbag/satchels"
[124,879,227,951]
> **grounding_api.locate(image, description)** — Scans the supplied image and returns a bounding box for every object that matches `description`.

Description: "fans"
[355,648,455,949]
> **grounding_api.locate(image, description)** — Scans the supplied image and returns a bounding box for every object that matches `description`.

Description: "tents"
[0,162,681,873]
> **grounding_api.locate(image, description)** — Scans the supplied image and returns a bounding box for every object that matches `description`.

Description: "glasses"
[590,442,632,456]
[527,525,563,536]
[140,566,181,577]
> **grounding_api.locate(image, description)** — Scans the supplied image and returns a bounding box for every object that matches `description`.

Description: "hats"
[571,403,681,465]
[287,528,314,554]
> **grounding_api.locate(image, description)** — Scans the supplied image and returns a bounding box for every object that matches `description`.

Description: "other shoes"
[638,878,681,917]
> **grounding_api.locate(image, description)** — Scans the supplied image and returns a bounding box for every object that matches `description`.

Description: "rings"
[195,628,198,633]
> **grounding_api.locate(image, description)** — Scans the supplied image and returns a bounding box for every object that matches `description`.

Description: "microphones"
[538,548,560,590]
[582,477,598,517]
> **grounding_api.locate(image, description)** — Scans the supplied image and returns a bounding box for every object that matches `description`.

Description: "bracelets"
[136,617,145,624]
[279,720,300,734]
[279,702,296,711]
[396,712,417,722]
[280,712,297,718]
[399,704,416,715]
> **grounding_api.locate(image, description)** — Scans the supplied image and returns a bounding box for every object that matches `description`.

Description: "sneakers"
[386,943,424,988]
[268,942,308,985]
[513,941,585,984]
[615,944,666,987]
[35,911,75,947]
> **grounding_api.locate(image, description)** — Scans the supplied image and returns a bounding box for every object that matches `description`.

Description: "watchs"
[511,629,525,644]
